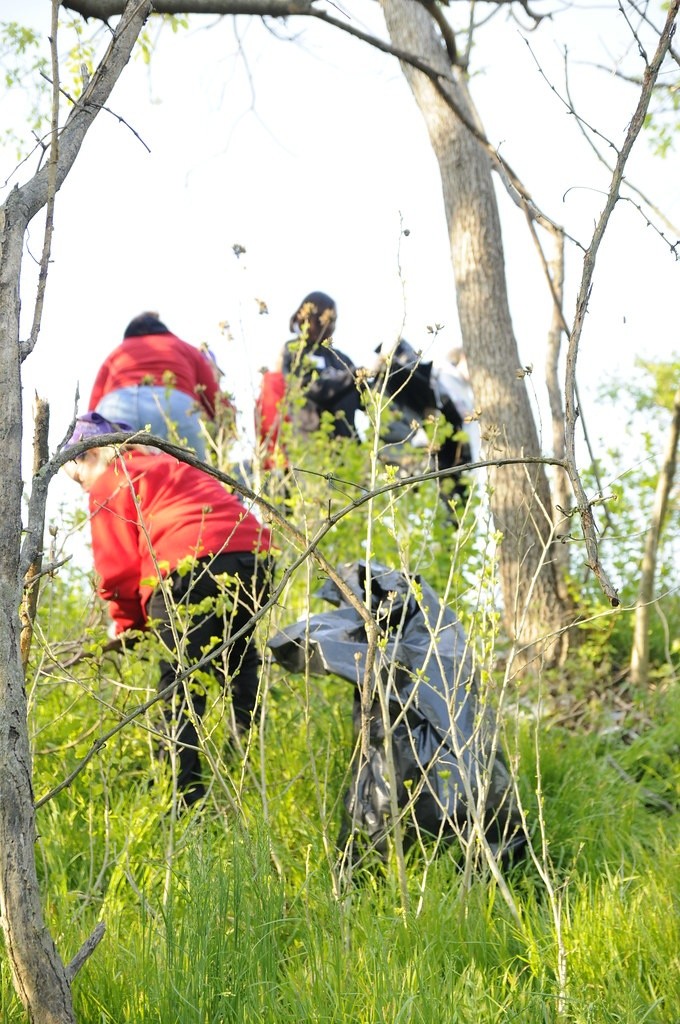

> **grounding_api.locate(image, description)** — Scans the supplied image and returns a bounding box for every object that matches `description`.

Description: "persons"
[283,292,366,446]
[254,372,294,521]
[62,412,275,817]
[89,312,235,464]
